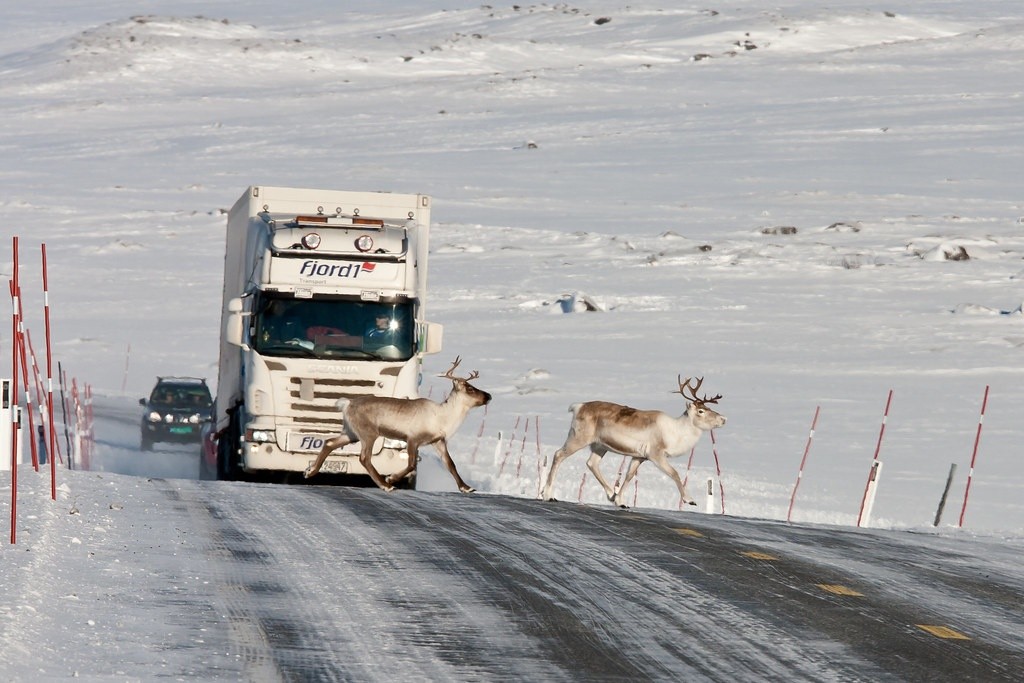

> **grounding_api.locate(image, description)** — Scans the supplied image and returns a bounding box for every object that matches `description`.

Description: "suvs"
[139,377,213,453]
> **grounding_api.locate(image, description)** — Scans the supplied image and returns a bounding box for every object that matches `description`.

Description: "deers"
[541,373,726,510]
[303,354,492,494]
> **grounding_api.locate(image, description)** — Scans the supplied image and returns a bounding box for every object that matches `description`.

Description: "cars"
[198,396,223,480]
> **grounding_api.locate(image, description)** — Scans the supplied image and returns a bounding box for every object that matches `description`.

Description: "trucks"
[218,187,444,490]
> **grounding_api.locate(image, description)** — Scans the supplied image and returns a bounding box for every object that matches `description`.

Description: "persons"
[270,312,305,341]
[366,311,401,344]
[190,389,204,404]
[162,392,175,403]
